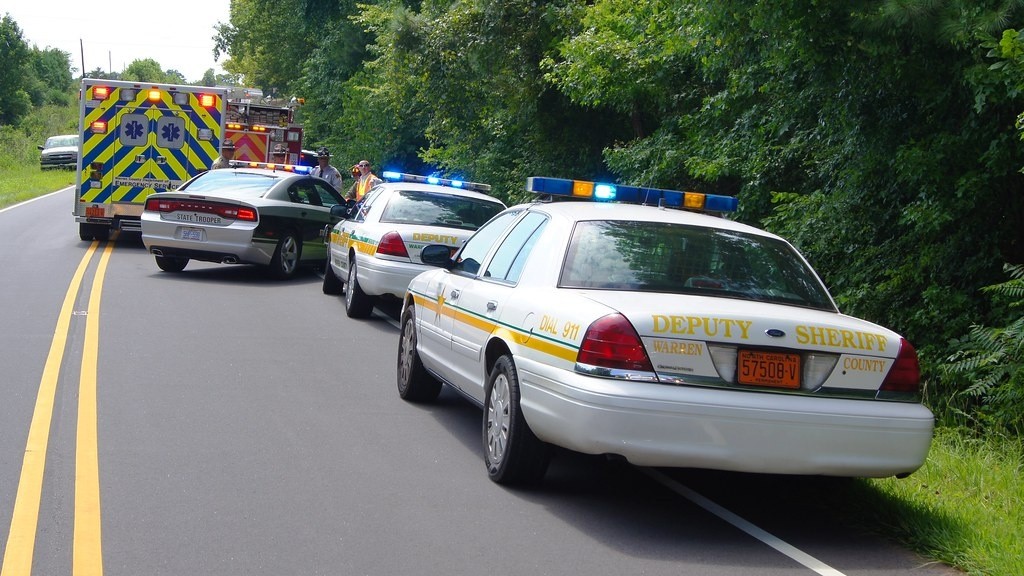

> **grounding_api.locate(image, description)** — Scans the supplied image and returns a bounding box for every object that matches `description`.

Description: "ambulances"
[71,78,228,241]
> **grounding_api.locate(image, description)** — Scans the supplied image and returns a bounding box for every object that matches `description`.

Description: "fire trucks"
[216,84,305,168]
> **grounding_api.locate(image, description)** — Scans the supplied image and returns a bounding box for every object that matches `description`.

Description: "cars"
[322,173,507,319]
[37,135,79,170]
[398,177,935,478]
[139,160,347,278]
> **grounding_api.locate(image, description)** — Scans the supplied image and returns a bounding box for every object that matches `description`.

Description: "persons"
[345,160,382,202]
[270,143,291,165]
[211,140,236,169]
[309,147,342,194]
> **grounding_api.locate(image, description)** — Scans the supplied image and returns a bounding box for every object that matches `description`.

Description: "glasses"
[359,164,369,167]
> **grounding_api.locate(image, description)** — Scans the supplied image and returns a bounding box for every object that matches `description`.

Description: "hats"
[217,139,239,148]
[269,144,286,154]
[313,147,334,158]
